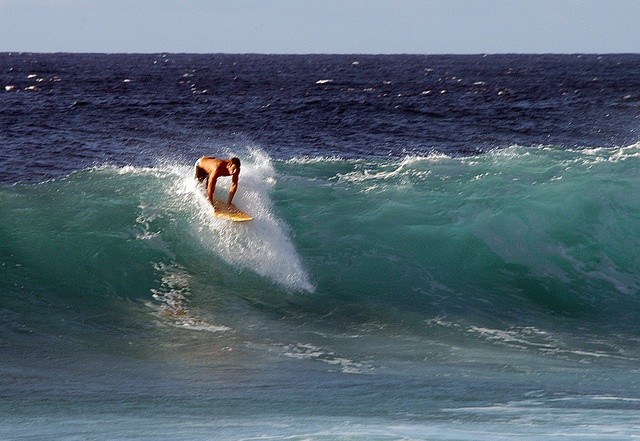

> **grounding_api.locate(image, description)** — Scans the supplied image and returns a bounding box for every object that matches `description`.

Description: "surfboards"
[207,198,252,223]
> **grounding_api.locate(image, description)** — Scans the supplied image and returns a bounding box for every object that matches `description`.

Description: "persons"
[194,155,241,214]
